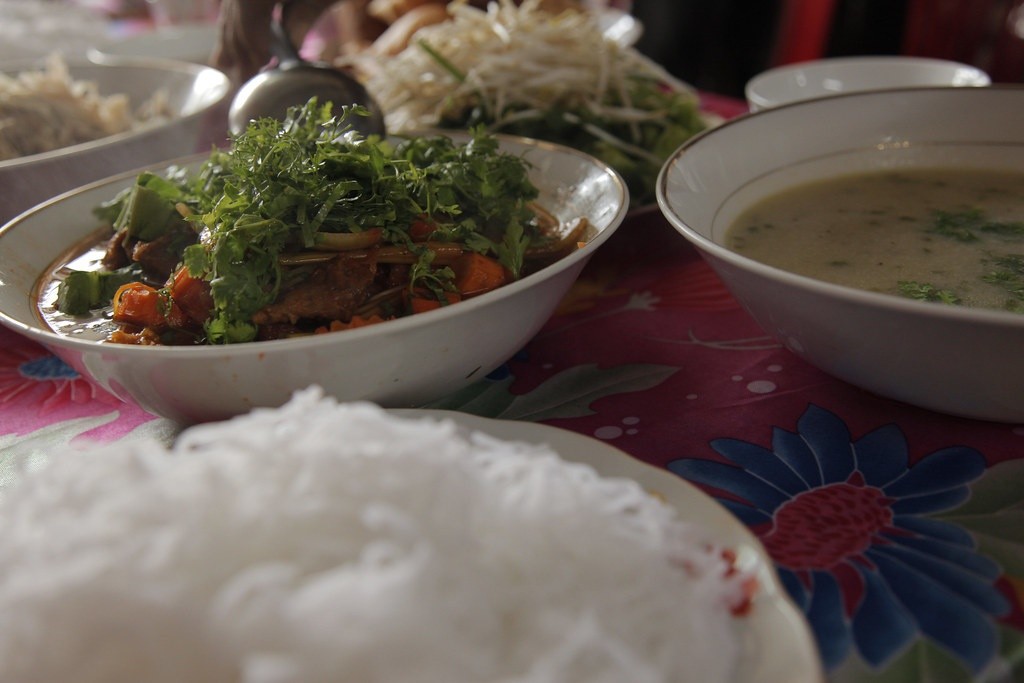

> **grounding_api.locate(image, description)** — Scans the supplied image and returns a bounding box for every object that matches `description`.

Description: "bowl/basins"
[1,43,230,227]
[656,86,1023,423]
[745,58,992,114]
[0,132,631,428]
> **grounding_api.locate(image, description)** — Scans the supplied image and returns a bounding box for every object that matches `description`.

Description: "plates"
[626,111,727,217]
[382,408,825,683]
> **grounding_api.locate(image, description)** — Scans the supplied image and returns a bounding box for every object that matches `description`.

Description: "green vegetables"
[58,98,541,344]
[894,205,1024,309]
[415,39,708,205]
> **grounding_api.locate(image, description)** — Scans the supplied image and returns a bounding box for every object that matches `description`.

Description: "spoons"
[225,25,388,146]
